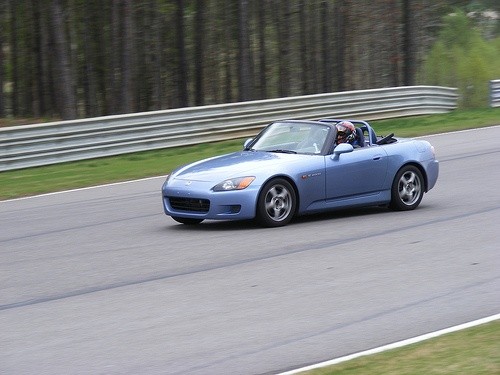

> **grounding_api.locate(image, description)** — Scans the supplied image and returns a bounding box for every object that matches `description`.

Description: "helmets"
[335,121,357,145]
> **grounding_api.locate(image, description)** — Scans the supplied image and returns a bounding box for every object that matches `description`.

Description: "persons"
[316,121,362,156]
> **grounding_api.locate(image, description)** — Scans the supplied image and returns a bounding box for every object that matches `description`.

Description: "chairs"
[356,127,364,147]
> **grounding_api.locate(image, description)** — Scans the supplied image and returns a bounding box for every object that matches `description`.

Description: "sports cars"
[161,117,439,226]
